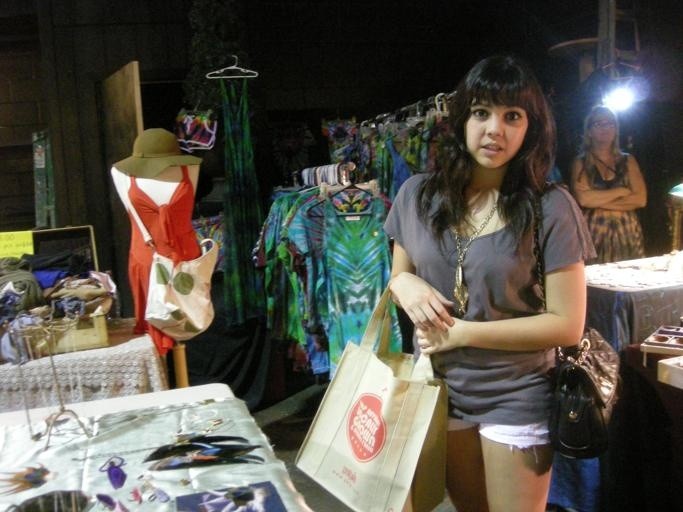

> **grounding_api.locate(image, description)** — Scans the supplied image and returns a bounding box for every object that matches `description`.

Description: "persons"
[569,106,647,265]
[383,54,598,512]
[111,128,203,357]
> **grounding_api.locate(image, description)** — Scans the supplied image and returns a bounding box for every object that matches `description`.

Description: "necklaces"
[589,150,614,180]
[451,204,498,313]
[588,148,624,177]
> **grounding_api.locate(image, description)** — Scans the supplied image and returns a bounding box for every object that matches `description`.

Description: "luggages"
[1,225,111,359]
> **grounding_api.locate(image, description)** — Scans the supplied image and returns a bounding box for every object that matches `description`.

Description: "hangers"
[182,88,218,121]
[294,158,374,218]
[205,54,259,80]
[351,90,453,143]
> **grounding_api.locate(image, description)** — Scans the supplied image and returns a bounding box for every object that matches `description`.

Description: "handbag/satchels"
[115,171,219,340]
[295,287,451,512]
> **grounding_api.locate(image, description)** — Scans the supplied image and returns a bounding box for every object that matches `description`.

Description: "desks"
[586,252,683,355]
[621,344,681,425]
[0,375,310,512]
[1,315,168,414]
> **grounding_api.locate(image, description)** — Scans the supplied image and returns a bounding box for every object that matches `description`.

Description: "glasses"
[531,180,629,456]
[589,119,618,128]
